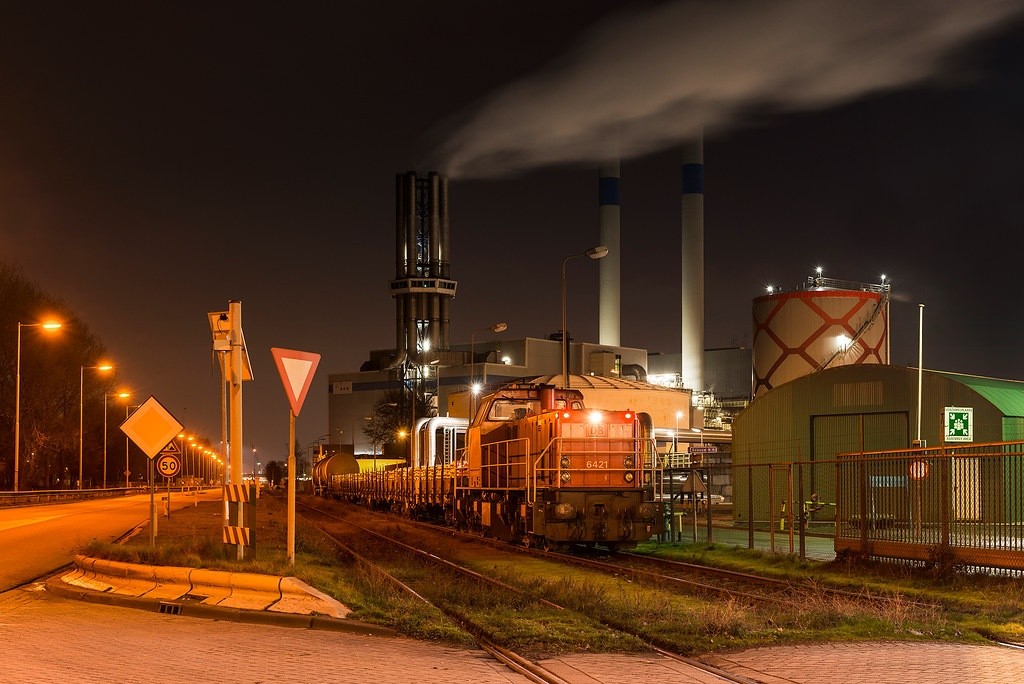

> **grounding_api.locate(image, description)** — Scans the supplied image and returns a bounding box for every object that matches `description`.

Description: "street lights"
[78,364,114,489]
[180,437,223,485]
[467,321,509,426]
[13,318,61,492]
[103,392,130,489]
[667,431,675,452]
[561,244,610,389]
[373,403,398,472]
[351,417,372,456]
[676,411,684,451]
[412,356,441,427]
[690,427,704,467]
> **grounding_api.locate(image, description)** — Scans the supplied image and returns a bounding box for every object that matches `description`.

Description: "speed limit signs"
[157,454,180,477]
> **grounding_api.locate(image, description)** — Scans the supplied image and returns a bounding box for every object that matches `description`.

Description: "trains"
[310,381,665,553]
